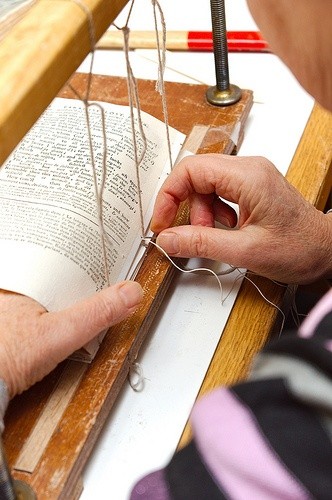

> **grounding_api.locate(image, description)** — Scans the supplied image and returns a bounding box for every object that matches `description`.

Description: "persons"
[0,1,332,499]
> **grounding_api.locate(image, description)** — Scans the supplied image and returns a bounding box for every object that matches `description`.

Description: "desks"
[175,97,331,451]
